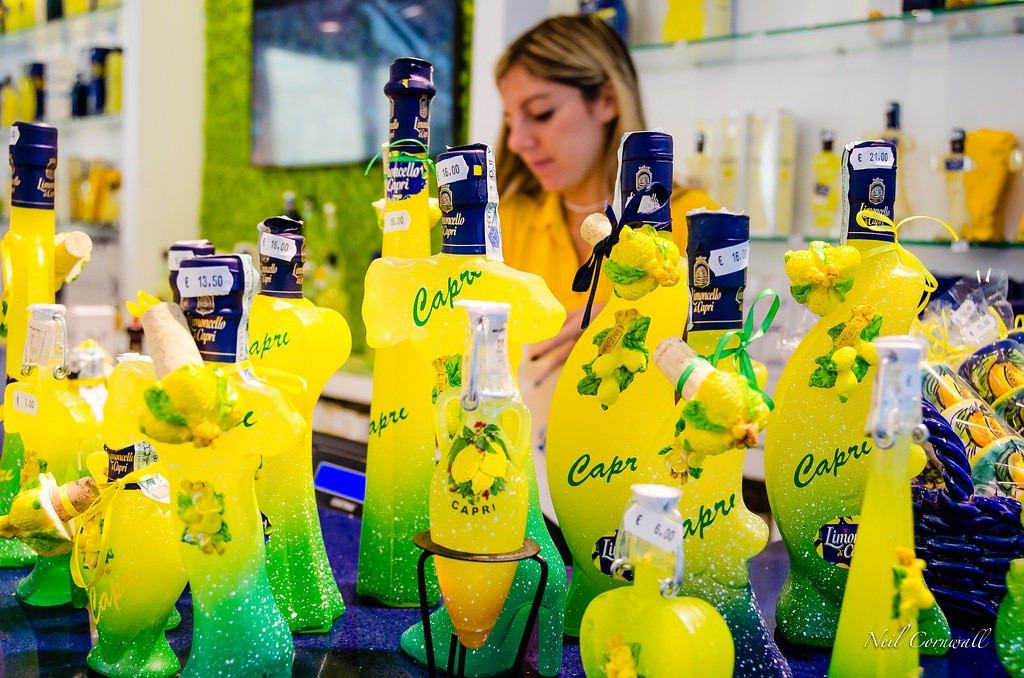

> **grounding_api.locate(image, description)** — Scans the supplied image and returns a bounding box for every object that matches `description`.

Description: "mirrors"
[249,0,462,173]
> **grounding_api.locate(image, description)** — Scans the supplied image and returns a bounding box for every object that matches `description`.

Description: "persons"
[493,15,724,389]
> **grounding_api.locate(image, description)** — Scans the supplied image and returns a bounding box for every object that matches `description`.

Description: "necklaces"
[564,198,615,214]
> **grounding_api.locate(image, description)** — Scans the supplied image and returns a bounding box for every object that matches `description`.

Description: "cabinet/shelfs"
[626,1,1024,252]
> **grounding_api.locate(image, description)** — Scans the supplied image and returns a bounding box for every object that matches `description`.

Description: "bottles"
[0,0,1024,678]
